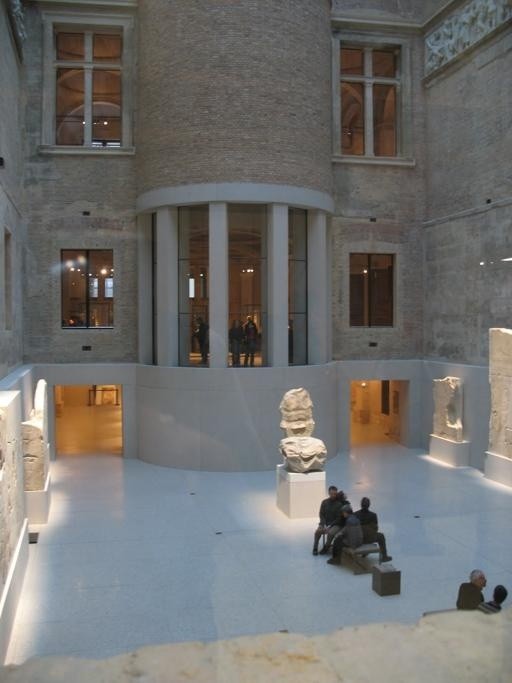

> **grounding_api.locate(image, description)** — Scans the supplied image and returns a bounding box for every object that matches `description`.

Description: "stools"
[373,563,401,597]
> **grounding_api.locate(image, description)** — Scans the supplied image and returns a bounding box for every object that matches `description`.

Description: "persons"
[455,568,487,610]
[337,490,353,527]
[312,485,342,556]
[242,315,259,367]
[193,317,209,365]
[477,584,507,614]
[288,319,293,363]
[355,496,392,562]
[229,319,242,365]
[326,503,363,565]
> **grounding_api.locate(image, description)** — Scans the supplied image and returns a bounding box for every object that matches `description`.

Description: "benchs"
[342,542,383,574]
